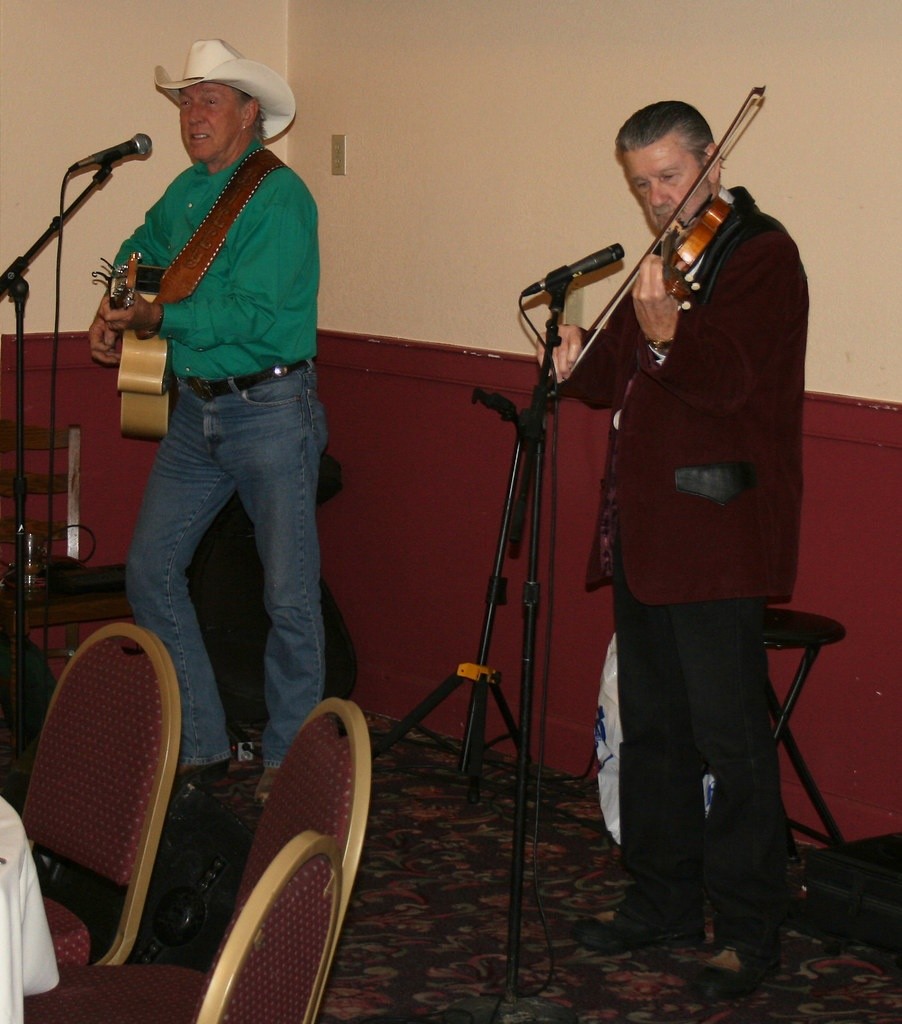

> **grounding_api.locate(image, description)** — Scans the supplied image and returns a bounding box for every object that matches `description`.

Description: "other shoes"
[570,896,707,951]
[252,767,280,807]
[689,951,769,1004]
[172,755,232,790]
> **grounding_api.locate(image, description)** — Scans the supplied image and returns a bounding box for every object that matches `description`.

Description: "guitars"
[108,250,175,443]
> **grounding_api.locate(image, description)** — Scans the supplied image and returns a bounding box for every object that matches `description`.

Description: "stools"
[759,607,847,849]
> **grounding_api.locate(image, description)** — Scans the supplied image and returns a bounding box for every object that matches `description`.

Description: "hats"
[155,39,296,140]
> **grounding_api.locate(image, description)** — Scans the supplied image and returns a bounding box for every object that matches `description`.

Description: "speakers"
[37,775,256,974]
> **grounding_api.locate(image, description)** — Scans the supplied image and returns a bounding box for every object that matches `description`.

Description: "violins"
[661,194,734,301]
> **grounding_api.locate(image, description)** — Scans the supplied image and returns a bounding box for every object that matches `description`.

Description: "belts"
[184,357,318,402]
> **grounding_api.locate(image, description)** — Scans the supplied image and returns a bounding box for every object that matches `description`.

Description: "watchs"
[136,303,164,340]
[646,337,674,356]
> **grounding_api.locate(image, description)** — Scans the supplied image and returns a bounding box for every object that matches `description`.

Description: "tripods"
[373,410,532,807]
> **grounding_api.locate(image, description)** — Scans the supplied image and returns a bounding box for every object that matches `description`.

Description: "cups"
[23,533,47,590]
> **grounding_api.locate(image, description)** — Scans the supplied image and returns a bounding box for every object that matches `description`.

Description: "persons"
[88,39,329,806]
[538,101,810,1000]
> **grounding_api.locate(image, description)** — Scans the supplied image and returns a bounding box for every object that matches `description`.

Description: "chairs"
[21,623,183,969]
[23,699,373,1024]
[193,827,344,1024]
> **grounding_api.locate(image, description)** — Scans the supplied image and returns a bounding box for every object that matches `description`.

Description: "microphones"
[68,133,152,173]
[520,243,625,297]
